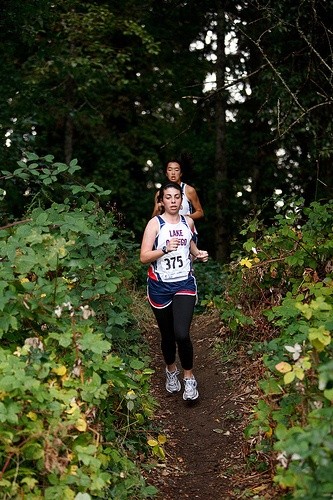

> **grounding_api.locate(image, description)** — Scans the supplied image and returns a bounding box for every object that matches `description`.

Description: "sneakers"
[165,365,181,393]
[183,375,199,400]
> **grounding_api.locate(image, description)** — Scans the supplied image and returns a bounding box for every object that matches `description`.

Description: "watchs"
[161,246,167,254]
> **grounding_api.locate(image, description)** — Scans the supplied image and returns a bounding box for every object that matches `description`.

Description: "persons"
[153,159,203,263]
[140,181,209,400]
